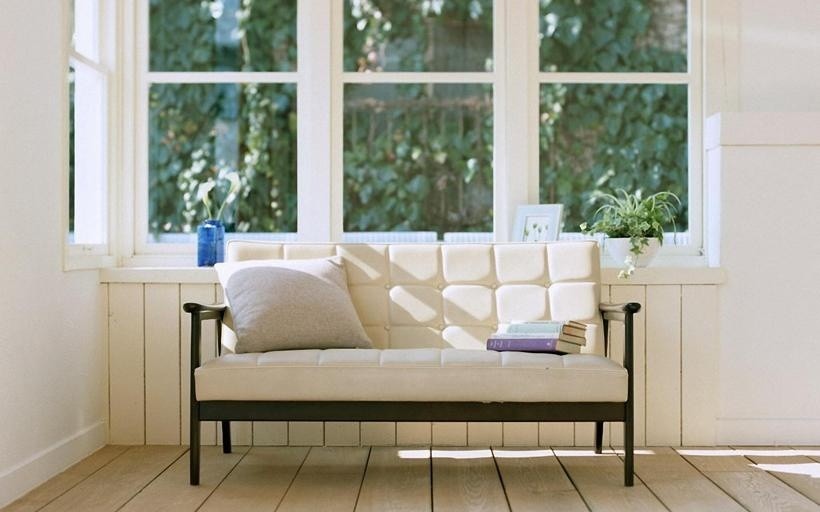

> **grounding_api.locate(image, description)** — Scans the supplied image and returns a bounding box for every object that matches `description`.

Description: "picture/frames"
[510,204,564,244]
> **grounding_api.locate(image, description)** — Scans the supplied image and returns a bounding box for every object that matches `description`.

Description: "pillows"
[213,256,374,355]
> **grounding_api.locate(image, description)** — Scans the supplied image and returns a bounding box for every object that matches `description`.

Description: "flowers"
[197,166,241,260]
[580,187,683,278]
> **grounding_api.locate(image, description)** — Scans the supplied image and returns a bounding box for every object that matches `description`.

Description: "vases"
[600,236,662,269]
[197,220,225,266]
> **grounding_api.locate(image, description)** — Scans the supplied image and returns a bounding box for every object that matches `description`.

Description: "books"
[486,320,586,355]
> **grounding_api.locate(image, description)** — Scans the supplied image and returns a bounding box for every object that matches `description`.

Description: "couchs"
[183,239,642,485]
